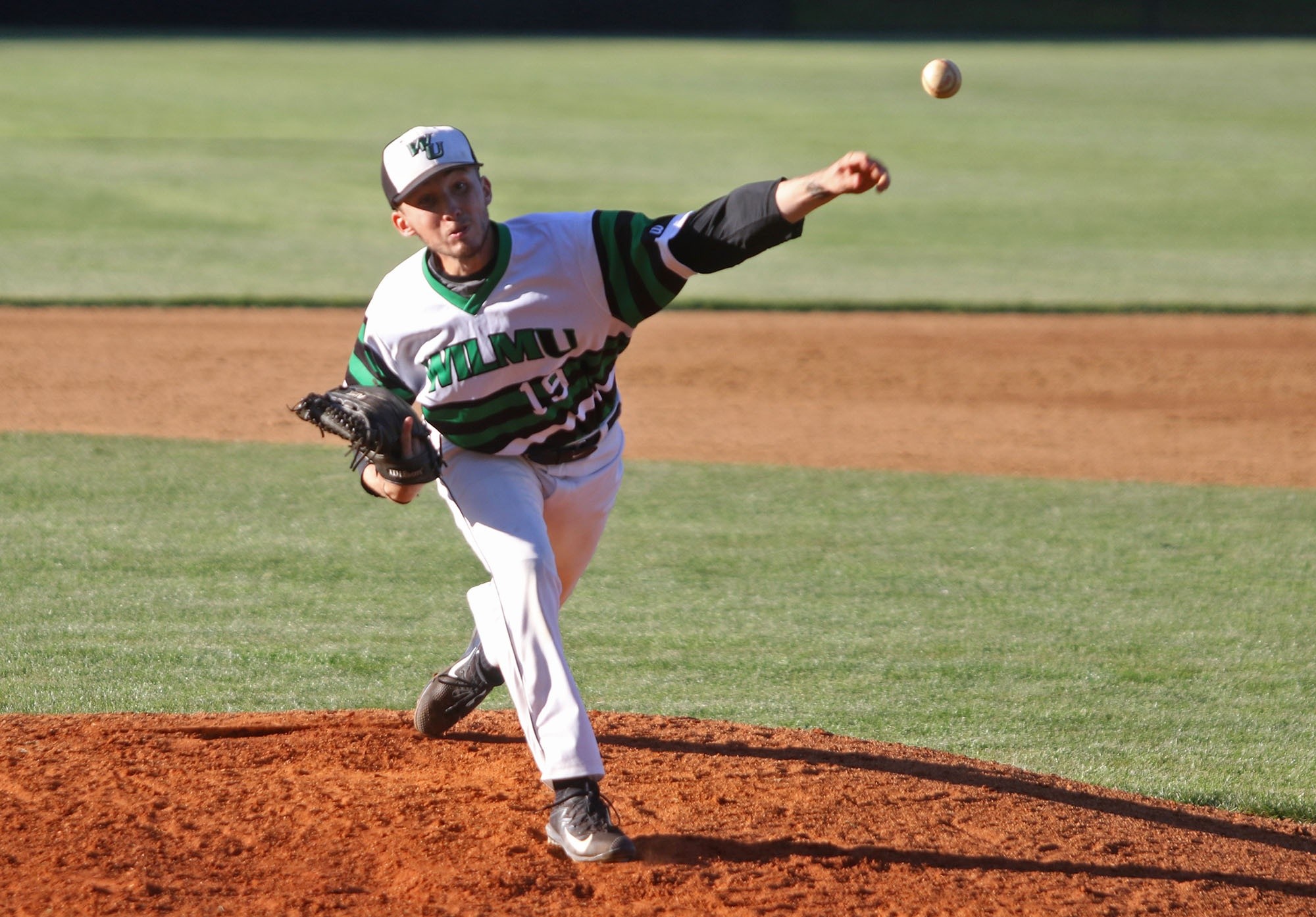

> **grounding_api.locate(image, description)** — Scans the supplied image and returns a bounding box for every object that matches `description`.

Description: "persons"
[340,124,893,863]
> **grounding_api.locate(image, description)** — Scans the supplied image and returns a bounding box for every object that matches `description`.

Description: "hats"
[381,125,484,210]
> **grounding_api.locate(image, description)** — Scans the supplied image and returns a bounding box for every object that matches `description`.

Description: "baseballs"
[922,58,961,98]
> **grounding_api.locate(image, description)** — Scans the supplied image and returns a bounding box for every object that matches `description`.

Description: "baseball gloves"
[295,383,444,485]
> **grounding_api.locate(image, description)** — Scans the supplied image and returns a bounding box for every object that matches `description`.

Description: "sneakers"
[414,625,504,736]
[544,787,635,861]
